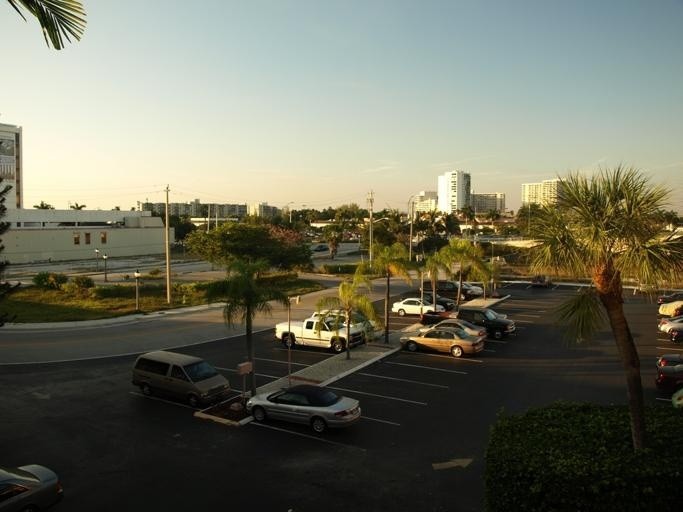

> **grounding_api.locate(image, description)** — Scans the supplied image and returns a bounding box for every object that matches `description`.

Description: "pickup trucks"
[274,315,363,350]
[418,306,515,341]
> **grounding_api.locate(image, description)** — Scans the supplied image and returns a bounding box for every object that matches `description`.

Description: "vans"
[309,309,381,342]
[132,352,230,404]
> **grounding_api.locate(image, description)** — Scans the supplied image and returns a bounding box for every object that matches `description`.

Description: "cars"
[0,464,63,511]
[531,276,551,288]
[399,329,484,357]
[391,278,482,313]
[656,289,682,409]
[417,319,487,340]
[448,307,508,319]
[313,245,328,252]
[245,385,361,434]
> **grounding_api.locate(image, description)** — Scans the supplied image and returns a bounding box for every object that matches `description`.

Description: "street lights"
[133,269,140,310]
[408,191,425,262]
[473,231,484,269]
[102,254,107,282]
[489,240,501,296]
[95,248,99,272]
[364,217,390,269]
